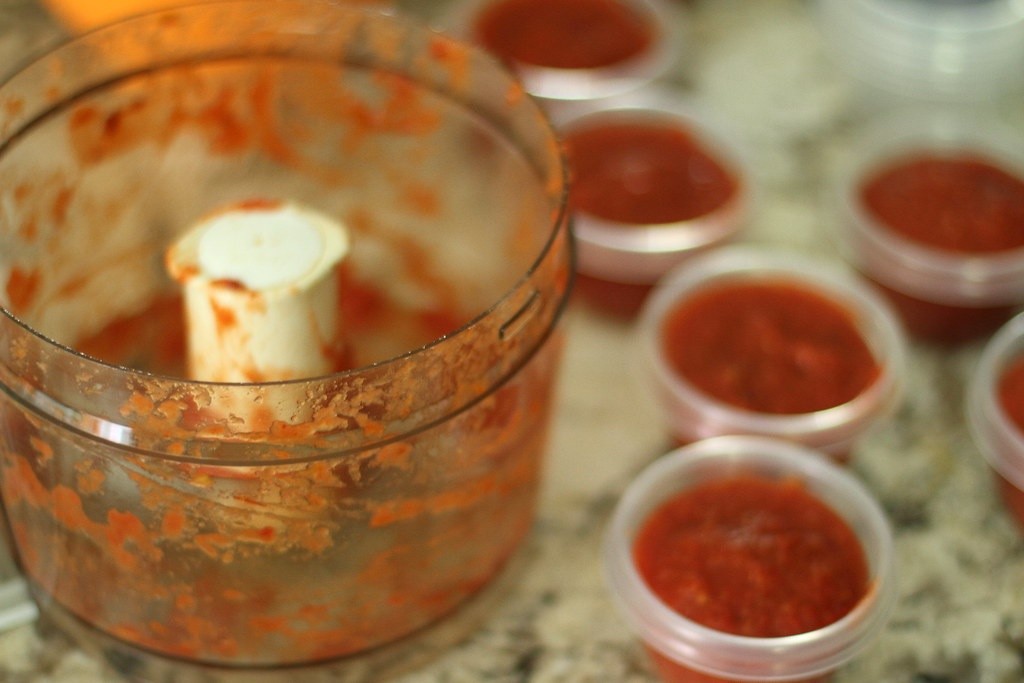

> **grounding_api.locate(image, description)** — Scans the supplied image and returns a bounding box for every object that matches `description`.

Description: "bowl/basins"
[470,0,676,125]
[605,435,897,683]
[559,109,749,318]
[635,244,908,467]
[0,0,568,683]
[835,0,1024,123]
[964,310,1024,529]
[832,117,1024,351]
